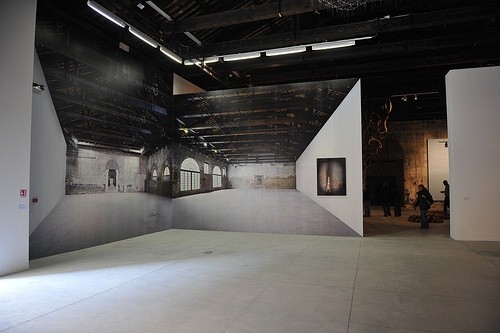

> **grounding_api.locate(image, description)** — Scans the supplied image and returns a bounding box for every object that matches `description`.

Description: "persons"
[382,183,401,216]
[441,180,450,212]
[364,186,370,217]
[414,185,432,228]
